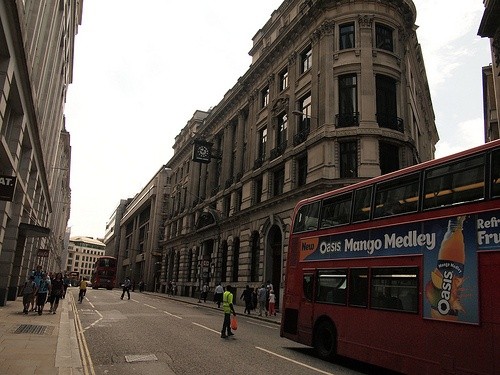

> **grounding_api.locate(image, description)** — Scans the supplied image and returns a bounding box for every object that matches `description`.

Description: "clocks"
[197,146,209,157]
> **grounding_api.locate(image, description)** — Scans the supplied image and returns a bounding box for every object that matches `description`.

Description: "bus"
[279,139,500,375]
[69,271,80,287]
[90,256,117,290]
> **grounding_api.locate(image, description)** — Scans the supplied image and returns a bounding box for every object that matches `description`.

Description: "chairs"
[382,296,403,309]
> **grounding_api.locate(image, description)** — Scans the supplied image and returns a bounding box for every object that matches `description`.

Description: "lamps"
[291,109,318,119]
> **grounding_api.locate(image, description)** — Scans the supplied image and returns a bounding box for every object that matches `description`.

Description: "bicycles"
[78,285,88,304]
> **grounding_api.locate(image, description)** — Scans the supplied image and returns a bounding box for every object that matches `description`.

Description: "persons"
[138,280,144,293]
[213,283,224,308]
[23,265,69,315]
[202,283,209,303]
[120,277,130,300]
[78,276,88,301]
[168,279,177,297]
[240,281,276,317]
[221,285,236,338]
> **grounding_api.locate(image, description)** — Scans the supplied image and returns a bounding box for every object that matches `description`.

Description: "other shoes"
[244,311,276,317]
[221,334,228,338]
[22,307,57,315]
[227,332,234,336]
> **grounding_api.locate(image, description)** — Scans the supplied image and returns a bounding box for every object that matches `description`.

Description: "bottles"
[430,219,465,320]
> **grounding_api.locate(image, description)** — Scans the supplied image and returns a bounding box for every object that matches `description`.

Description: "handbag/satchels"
[230,316,237,330]
